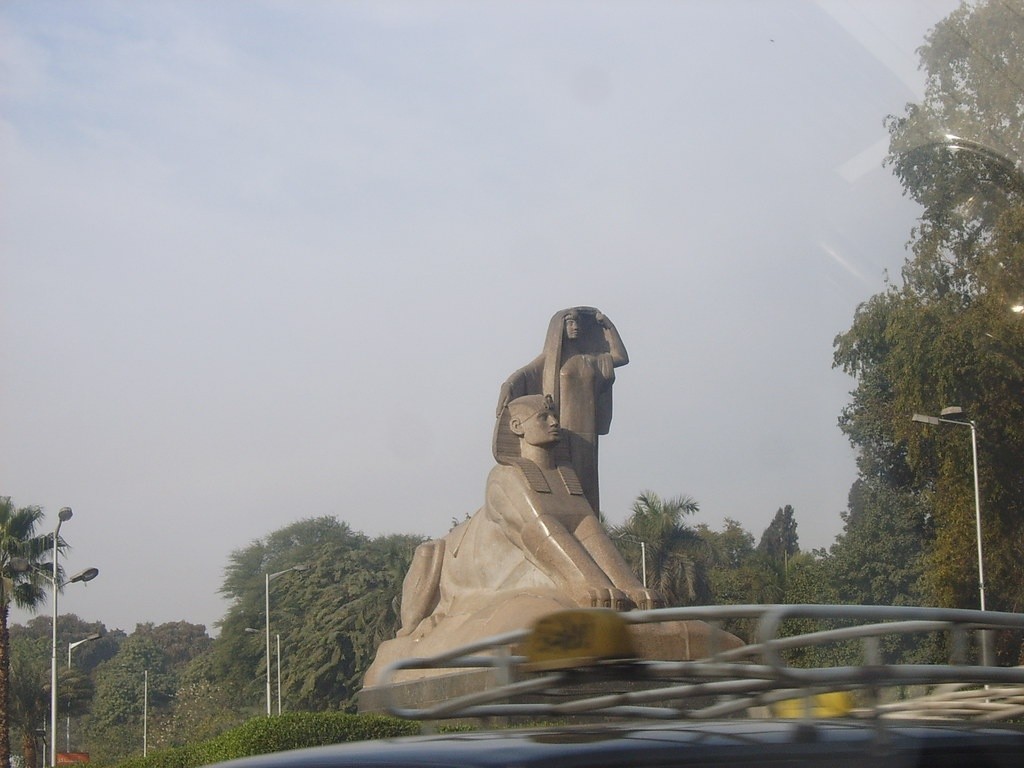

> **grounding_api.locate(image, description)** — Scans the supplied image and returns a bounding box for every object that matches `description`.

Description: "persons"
[484,306,668,611]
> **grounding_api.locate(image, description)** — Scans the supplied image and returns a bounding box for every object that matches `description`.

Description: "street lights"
[245,627,283,717]
[265,563,309,718]
[33,707,48,768]
[66,633,102,753]
[913,406,991,703]
[10,505,100,768]
[610,535,647,589]
[122,664,149,757]
